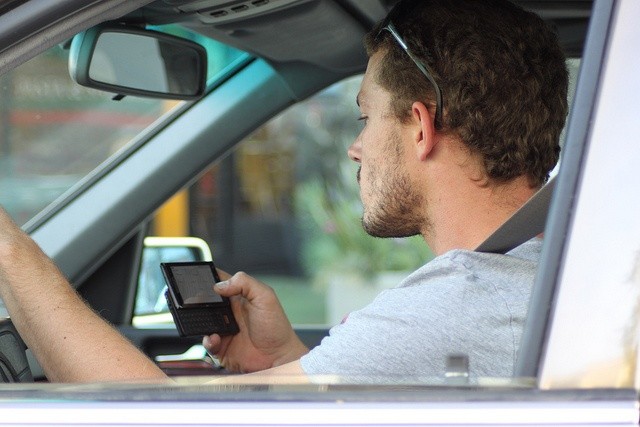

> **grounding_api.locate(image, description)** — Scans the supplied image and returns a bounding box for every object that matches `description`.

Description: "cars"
[0,0,640,426]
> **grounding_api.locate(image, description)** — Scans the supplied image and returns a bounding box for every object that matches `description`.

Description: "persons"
[0,0,568,384]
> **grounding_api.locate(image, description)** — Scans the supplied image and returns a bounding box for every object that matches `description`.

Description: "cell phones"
[159,259,242,340]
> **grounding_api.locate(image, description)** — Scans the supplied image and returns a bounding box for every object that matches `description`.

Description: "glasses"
[370,4,444,130]
